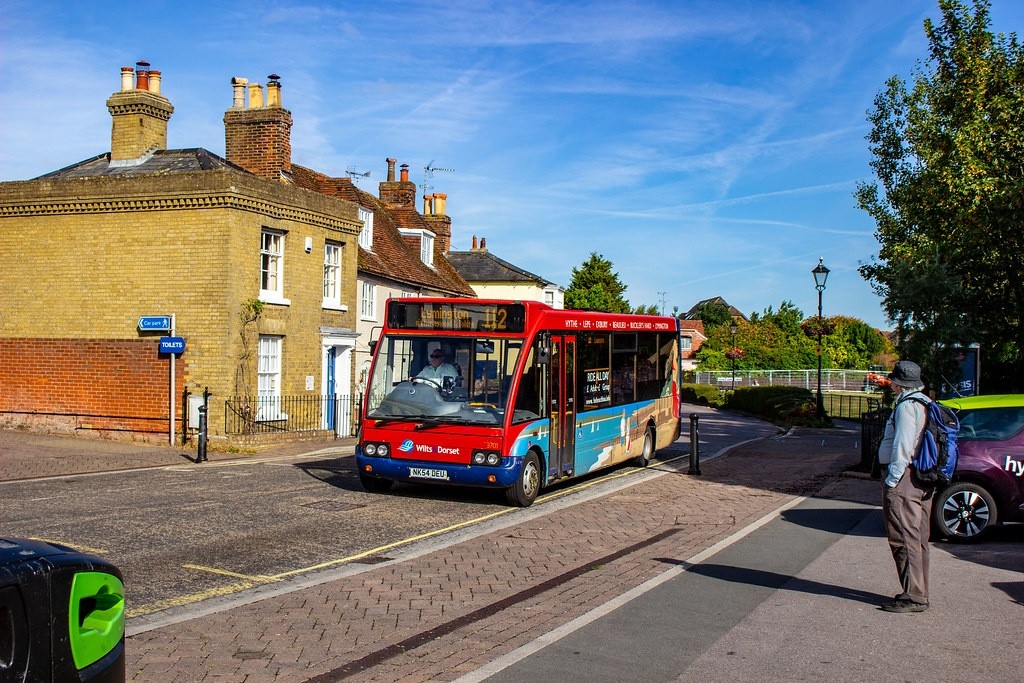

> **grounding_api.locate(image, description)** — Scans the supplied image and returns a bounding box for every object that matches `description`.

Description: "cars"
[0,536,125,683]
[936,394,1024,543]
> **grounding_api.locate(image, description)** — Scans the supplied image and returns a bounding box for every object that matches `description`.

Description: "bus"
[356,298,683,508]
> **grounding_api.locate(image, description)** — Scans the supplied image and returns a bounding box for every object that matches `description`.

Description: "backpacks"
[889,395,961,501]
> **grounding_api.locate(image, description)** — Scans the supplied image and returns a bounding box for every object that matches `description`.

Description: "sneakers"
[882,593,931,613]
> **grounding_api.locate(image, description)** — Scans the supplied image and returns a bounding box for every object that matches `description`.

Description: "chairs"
[451,363,463,388]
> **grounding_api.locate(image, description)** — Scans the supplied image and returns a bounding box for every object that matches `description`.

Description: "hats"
[888,361,923,388]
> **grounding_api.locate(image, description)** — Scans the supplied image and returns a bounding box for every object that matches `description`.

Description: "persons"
[878,361,936,612]
[408,348,459,390]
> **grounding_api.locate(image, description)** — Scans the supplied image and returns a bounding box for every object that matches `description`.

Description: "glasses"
[430,354,444,359]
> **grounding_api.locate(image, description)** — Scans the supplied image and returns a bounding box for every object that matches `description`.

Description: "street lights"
[812,256,831,413]
[728,320,740,392]
